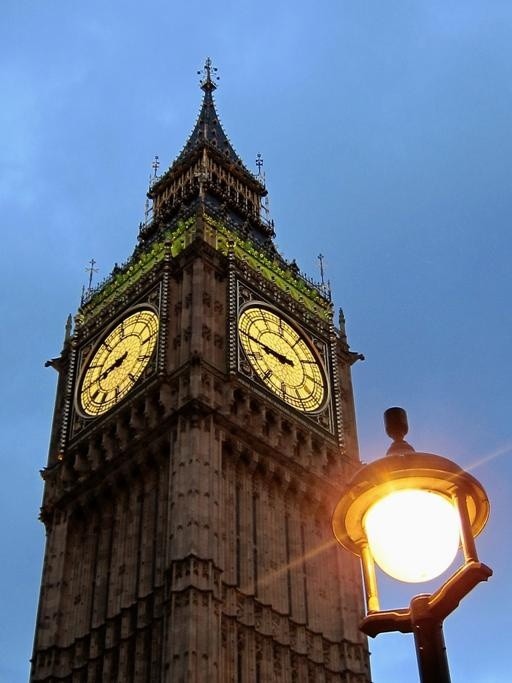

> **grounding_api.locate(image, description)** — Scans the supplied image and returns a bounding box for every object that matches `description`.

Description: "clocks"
[234,292,330,416]
[67,302,172,420]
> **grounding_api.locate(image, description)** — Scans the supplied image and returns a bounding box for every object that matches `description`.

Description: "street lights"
[331,406,493,682]
